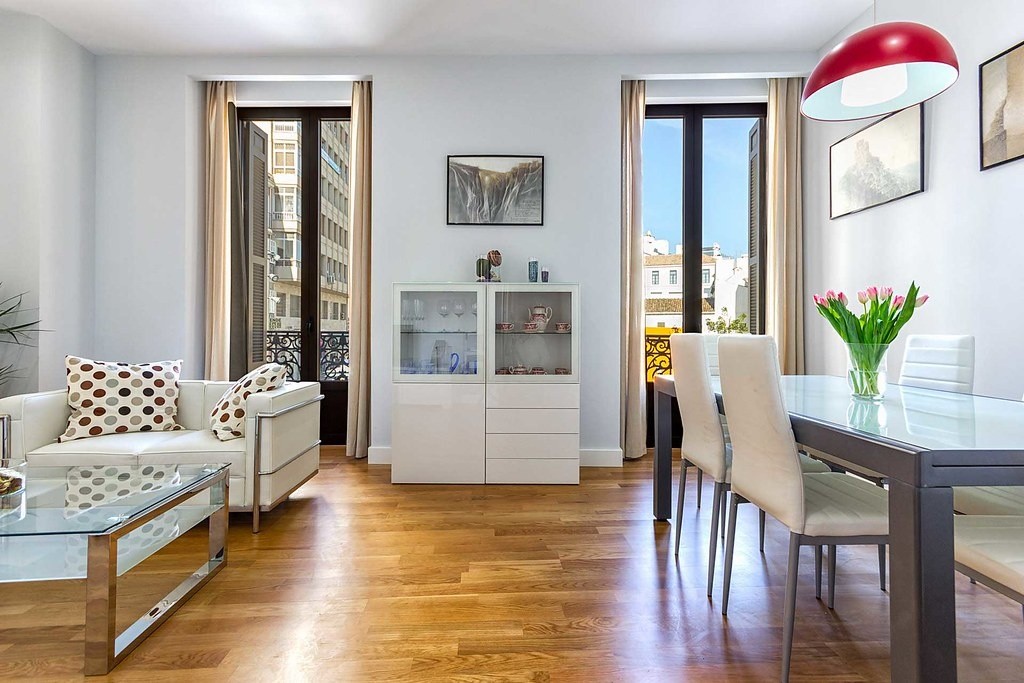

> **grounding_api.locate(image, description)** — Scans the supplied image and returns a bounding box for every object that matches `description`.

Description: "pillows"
[57,354,186,443]
[210,362,288,441]
[64,464,182,558]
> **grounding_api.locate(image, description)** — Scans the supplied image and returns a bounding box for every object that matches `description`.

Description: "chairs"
[670,332,1024,683]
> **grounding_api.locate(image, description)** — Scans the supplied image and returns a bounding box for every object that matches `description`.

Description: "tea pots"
[528,303,552,332]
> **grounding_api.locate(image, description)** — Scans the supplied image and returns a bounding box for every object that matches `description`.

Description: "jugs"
[436,345,459,374]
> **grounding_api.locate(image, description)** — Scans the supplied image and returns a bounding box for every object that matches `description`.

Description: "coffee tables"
[0,461,232,677]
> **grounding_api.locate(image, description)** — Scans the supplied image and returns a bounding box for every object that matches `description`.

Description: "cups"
[524,322,540,332]
[476,254,490,281]
[528,256,539,283]
[556,323,571,332]
[496,365,566,375]
[541,265,549,282]
[498,323,515,331]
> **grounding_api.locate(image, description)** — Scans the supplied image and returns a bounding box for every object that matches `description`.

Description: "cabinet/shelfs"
[391,282,580,486]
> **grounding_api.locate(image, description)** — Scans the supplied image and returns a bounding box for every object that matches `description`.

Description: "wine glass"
[401,299,477,332]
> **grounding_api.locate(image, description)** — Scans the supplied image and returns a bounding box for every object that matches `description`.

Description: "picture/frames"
[829,102,924,219]
[447,154,545,226]
[979,41,1024,171]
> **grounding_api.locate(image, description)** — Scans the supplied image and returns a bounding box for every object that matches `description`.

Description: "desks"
[653,375,1024,683]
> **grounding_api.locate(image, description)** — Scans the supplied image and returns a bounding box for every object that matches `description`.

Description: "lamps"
[799,0,959,122]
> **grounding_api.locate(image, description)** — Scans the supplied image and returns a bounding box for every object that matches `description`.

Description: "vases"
[844,342,888,399]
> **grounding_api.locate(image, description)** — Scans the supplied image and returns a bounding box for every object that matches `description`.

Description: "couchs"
[1,379,325,532]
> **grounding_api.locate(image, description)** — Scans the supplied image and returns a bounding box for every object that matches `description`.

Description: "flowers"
[813,280,929,395]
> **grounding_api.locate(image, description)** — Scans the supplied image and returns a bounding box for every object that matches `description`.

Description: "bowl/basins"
[0,458,28,497]
[0,491,26,528]
